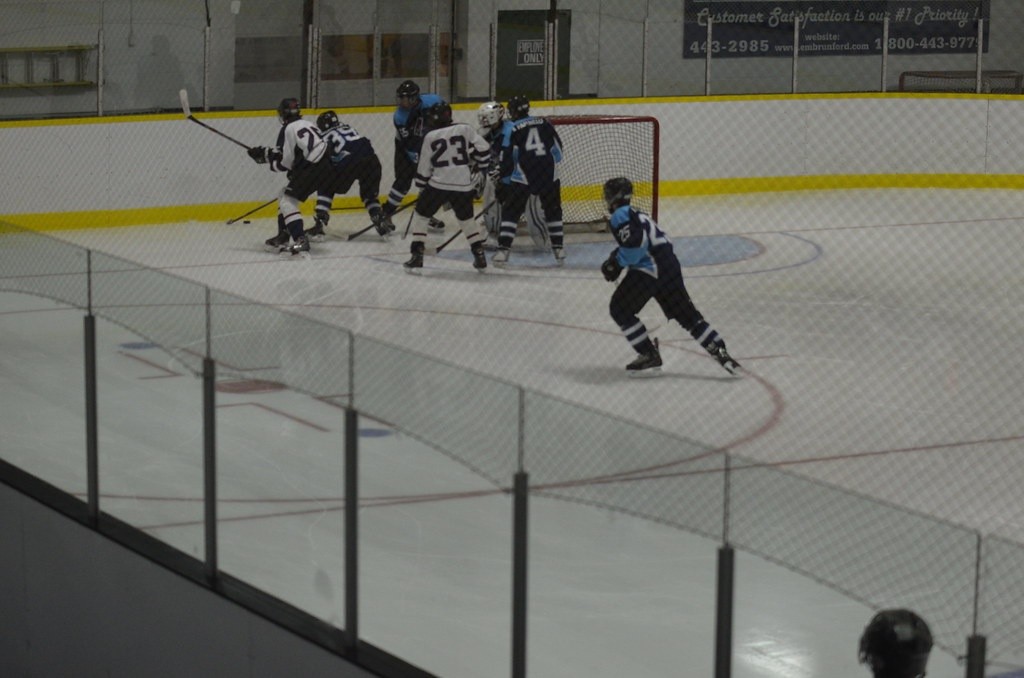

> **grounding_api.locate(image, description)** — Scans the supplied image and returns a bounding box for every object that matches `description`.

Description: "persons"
[381,80,452,231]
[134,34,202,108]
[302,111,391,236]
[248,98,331,254]
[490,96,566,262]
[858,609,933,678]
[403,103,491,268]
[601,177,742,375]
[468,101,551,251]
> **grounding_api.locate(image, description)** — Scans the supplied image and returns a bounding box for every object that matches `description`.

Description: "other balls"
[244,220,250,223]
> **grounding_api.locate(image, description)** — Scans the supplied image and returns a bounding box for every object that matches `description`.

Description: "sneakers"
[625,338,664,377]
[279,236,312,260]
[554,248,567,267]
[380,204,396,232]
[491,247,511,268]
[472,246,488,273]
[305,213,330,242]
[708,342,748,377]
[428,217,445,235]
[369,207,391,242]
[404,243,425,275]
[264,233,289,252]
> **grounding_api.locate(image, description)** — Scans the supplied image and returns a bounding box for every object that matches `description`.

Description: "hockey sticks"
[227,197,279,225]
[607,265,659,352]
[326,197,421,242]
[425,199,498,255]
[179,88,253,151]
[399,190,421,240]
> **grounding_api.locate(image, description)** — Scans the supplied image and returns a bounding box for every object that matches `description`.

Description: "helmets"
[278,98,301,119]
[396,80,419,104]
[477,101,504,126]
[506,95,531,119]
[317,110,339,131]
[858,608,935,677]
[428,101,452,128]
[603,178,633,213]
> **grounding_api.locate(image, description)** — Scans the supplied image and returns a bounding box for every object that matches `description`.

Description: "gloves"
[247,146,281,163]
[600,246,624,281]
